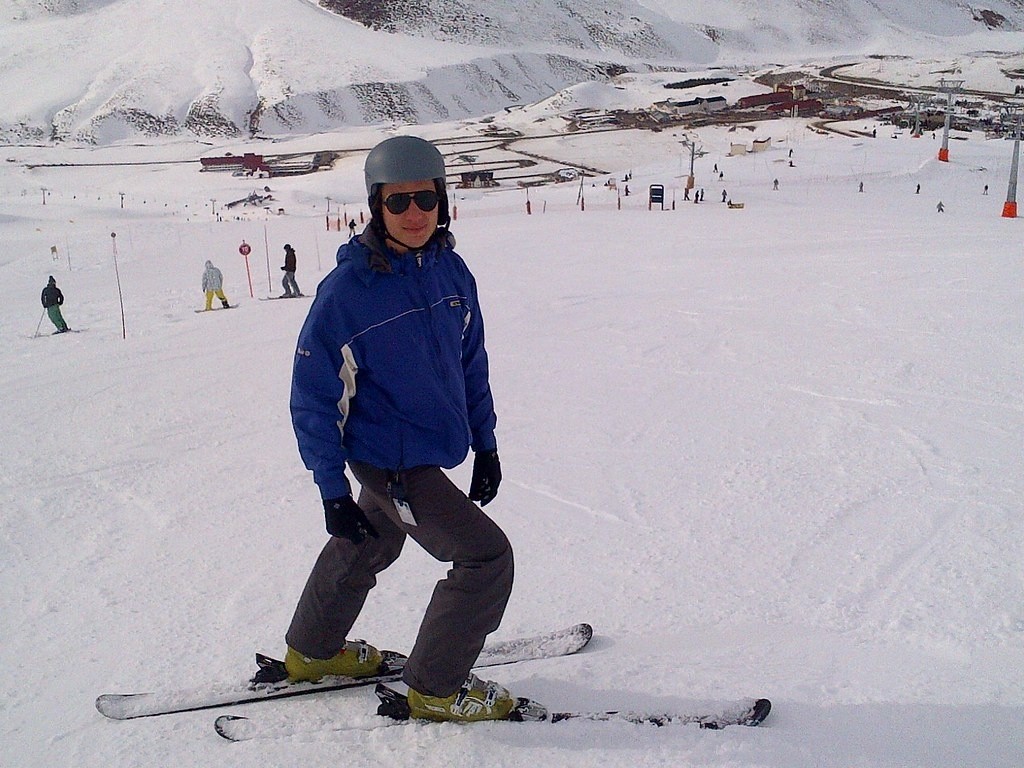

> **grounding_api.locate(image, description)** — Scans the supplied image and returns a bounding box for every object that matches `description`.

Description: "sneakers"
[284,640,383,684]
[406,672,513,723]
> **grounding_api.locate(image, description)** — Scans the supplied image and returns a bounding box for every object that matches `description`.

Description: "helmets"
[365,135,449,227]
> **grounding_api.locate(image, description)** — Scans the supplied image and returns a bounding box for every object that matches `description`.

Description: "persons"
[917,184,920,193]
[695,188,704,203]
[873,129,877,138]
[932,133,935,139]
[284,135,515,720]
[983,185,988,194]
[773,178,778,190]
[722,190,727,202]
[683,188,690,201]
[202,261,229,310]
[41,276,67,332]
[937,201,944,212]
[859,182,863,192]
[625,170,632,181]
[788,149,793,166]
[625,185,630,196]
[714,163,723,180]
[349,219,356,238]
[281,244,301,295]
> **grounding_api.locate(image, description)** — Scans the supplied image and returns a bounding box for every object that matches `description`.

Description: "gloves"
[324,496,380,543]
[468,448,500,506]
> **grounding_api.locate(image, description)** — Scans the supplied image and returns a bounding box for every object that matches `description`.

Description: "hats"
[48,276,56,284]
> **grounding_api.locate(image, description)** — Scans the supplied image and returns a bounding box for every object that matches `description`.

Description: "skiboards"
[95,619,774,742]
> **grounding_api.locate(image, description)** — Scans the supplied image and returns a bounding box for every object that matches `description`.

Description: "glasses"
[382,190,443,215]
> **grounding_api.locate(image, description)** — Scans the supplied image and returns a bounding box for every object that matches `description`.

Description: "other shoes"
[55,326,69,333]
